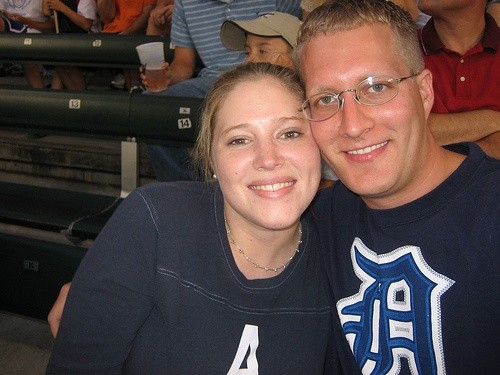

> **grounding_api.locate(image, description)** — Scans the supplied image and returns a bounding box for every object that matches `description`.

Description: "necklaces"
[224,217,303,271]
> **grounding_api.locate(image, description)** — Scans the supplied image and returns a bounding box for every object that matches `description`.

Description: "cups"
[136,42,166,92]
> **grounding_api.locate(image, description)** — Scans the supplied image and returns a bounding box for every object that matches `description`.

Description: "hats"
[220,11,304,50]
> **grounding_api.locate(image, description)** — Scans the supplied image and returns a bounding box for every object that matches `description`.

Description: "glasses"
[297,72,421,121]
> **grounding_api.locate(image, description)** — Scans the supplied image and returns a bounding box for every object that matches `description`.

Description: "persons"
[0,0,174,138]
[220,11,303,71]
[300,0,500,160]
[47,0,500,375]
[45,62,361,375]
[141,0,303,182]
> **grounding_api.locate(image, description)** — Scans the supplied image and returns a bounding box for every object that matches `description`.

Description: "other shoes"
[111,77,126,89]
